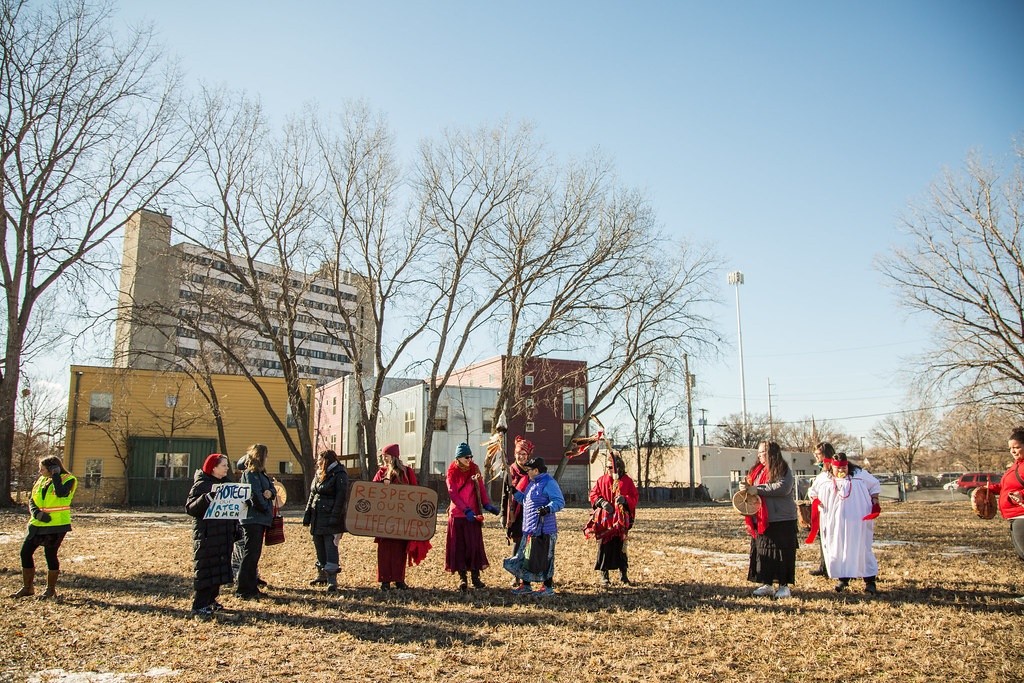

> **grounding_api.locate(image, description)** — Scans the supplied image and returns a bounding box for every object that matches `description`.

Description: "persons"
[809,442,836,579]
[9,454,78,598]
[234,444,277,600]
[445,442,500,591]
[185,453,242,616]
[506,457,565,598]
[739,440,799,598]
[302,449,349,593]
[808,452,882,595]
[500,434,536,586]
[371,444,419,591]
[589,454,639,587]
[979,426,1024,605]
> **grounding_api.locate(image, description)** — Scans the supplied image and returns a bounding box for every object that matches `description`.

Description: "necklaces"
[832,475,852,498]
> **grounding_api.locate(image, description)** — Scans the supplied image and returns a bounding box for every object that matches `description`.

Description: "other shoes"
[834,582,849,592]
[865,583,876,593]
[809,567,826,576]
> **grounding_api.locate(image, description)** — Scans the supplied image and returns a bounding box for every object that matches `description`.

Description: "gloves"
[205,491,217,502]
[537,506,551,516]
[599,499,613,512]
[485,505,502,516]
[464,507,475,521]
[36,512,51,523]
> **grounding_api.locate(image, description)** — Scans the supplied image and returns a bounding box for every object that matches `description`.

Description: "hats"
[455,442,474,458]
[203,454,223,476]
[614,495,625,505]
[381,444,399,458]
[514,435,534,455]
[523,457,545,468]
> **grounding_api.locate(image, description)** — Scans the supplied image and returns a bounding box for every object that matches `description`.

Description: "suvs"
[956,473,1003,498]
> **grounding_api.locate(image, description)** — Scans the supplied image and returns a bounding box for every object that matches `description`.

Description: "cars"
[872,472,966,491]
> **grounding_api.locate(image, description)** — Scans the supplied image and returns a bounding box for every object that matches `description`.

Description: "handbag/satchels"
[265,495,285,545]
[524,514,550,574]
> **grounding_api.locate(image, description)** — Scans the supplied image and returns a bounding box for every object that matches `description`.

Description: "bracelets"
[872,497,878,500]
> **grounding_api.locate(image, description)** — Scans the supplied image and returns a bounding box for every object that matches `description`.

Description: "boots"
[9,568,35,597]
[38,570,59,598]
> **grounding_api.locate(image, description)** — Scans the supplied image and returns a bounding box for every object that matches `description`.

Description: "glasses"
[606,466,613,469]
[464,455,473,459]
[525,469,531,474]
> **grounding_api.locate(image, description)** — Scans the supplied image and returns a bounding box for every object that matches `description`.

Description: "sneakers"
[753,584,775,595]
[775,585,791,599]
[194,575,632,614]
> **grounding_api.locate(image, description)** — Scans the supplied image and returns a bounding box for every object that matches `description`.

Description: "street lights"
[861,437,865,448]
[728,272,747,448]
